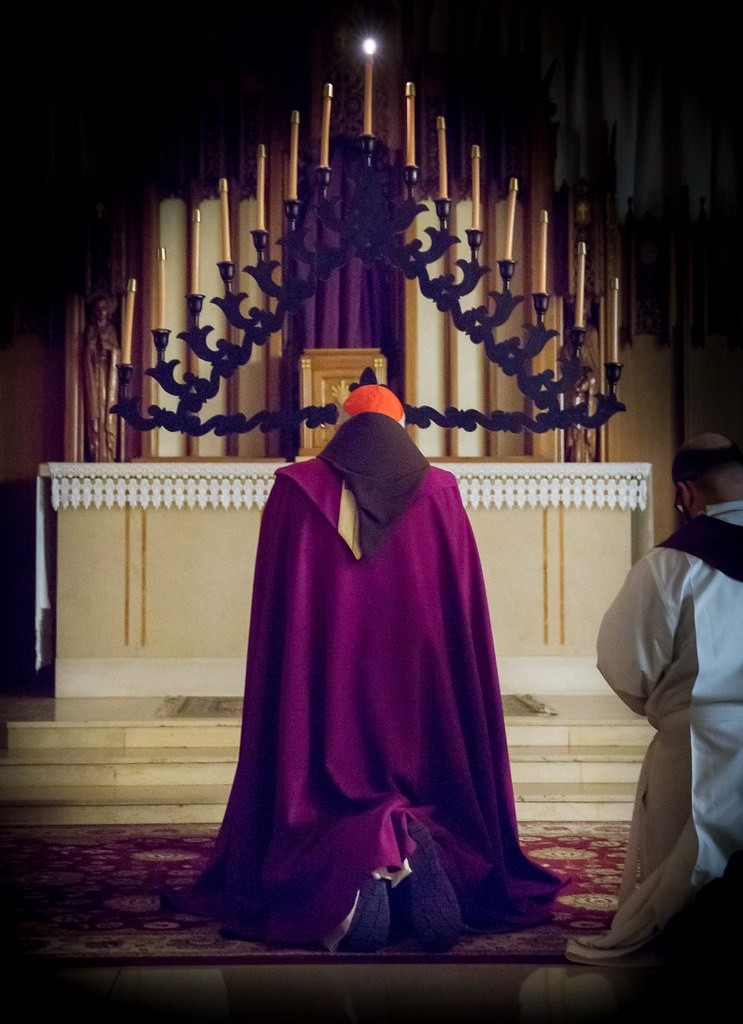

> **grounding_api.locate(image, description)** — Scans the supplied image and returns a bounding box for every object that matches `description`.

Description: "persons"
[566,433,743,967]
[176,384,560,952]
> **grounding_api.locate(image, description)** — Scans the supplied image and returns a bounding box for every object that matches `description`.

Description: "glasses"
[673,487,691,517]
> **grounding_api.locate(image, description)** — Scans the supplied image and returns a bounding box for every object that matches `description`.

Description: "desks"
[33,461,654,697]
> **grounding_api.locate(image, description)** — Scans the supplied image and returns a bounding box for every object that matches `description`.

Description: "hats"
[343,383,405,425]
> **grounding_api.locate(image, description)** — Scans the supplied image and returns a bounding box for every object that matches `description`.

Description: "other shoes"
[400,821,464,953]
[344,871,392,957]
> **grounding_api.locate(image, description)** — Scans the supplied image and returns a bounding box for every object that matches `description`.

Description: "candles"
[575,242,587,326]
[471,144,480,228]
[437,117,448,198]
[190,208,202,293]
[121,279,137,364]
[287,111,300,200]
[217,178,231,260]
[319,83,333,167]
[503,178,519,259]
[154,247,167,329]
[609,276,619,362]
[404,82,416,164]
[256,144,267,231]
[536,211,547,293]
[363,38,375,133]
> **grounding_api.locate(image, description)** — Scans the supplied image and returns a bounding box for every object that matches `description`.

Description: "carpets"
[0,820,643,968]
